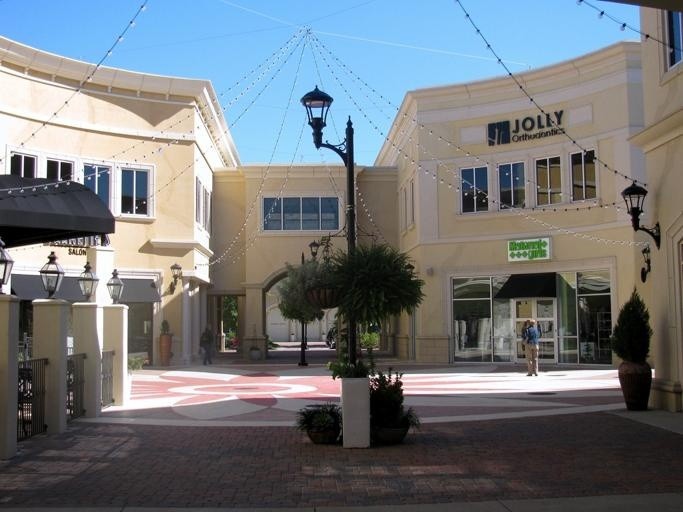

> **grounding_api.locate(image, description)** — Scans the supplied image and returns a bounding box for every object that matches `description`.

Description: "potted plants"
[369,367,420,444]
[609,286,654,411]
[250,346,261,360]
[276,260,348,323]
[297,400,342,443]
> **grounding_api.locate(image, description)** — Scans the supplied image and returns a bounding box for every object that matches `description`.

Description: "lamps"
[641,244,651,283]
[169,263,182,294]
[621,183,661,249]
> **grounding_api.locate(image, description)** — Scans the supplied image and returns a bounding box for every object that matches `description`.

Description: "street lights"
[299,239,319,368]
[299,82,360,369]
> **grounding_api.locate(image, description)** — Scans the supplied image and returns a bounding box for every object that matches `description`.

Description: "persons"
[522,318,541,376]
[200,323,214,365]
